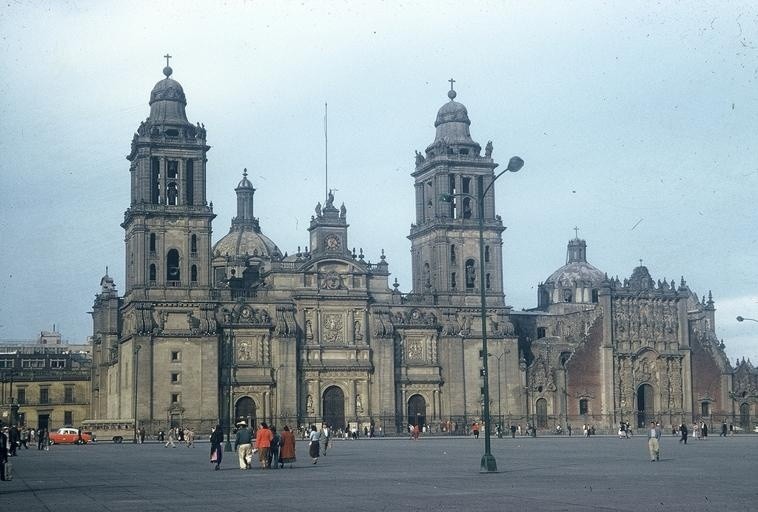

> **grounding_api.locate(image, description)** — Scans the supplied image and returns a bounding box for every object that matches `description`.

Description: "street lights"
[735,315,758,325]
[476,153,526,476]
[223,298,247,453]
[132,343,141,444]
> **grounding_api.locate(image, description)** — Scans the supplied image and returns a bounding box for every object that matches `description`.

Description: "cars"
[49,426,92,445]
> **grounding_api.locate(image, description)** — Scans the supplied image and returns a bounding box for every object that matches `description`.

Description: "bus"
[80,418,138,444]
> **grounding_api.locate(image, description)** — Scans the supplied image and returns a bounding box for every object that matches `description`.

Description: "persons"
[267,424,281,469]
[278,425,296,468]
[254,422,274,470]
[134,422,217,449]
[233,420,254,471]
[308,424,321,464]
[207,424,224,470]
[0,419,89,480]
[318,422,332,457]
[647,421,662,464]
